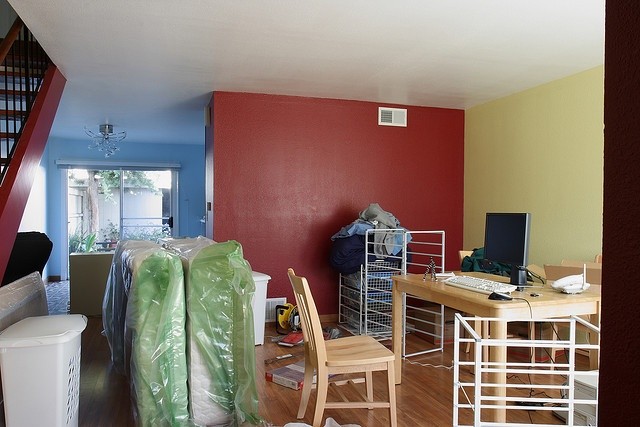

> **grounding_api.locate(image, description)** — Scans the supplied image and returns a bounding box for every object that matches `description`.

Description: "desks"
[391,270,603,424]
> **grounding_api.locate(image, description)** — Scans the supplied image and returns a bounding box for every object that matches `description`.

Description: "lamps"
[84,124,126,158]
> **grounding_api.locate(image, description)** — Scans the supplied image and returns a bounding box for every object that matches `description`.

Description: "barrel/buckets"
[0,313,89,427]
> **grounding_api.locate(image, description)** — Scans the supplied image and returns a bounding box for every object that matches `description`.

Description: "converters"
[515,400,544,406]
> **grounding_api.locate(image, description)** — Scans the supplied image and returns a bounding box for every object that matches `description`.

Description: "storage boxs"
[414,305,465,344]
[250,269,271,347]
[507,321,569,363]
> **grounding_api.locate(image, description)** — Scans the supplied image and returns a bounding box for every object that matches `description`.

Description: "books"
[265,358,339,390]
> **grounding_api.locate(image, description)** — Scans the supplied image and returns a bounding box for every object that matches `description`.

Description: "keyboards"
[445,276,517,295]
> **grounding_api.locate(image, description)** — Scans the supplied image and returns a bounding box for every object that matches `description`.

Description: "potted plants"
[102,219,119,248]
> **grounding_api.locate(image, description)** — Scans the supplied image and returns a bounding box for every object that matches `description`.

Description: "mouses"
[488,292,513,300]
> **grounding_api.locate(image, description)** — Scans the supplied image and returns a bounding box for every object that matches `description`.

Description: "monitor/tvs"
[485,212,534,282]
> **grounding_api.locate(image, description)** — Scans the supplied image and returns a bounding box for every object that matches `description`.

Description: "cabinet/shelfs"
[453,312,600,427]
[338,228,445,360]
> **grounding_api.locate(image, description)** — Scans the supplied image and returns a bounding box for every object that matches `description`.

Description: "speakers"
[510,269,527,291]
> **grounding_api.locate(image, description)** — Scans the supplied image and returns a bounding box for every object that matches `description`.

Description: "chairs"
[544,264,603,370]
[286,267,397,427]
[459,250,473,267]
